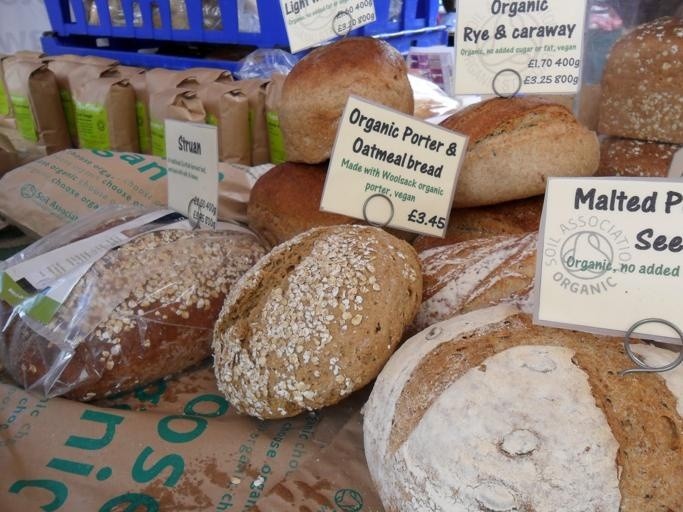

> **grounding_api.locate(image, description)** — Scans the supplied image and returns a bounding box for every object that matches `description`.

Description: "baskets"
[44,0,450,74]
[43,1,450,74]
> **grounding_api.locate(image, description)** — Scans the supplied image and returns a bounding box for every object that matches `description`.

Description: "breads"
[1,16,682,512]
